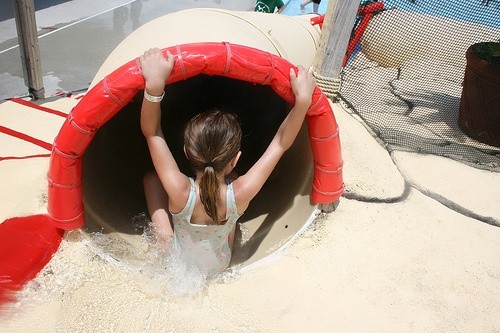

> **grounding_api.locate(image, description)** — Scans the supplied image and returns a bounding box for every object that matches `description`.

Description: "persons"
[300,0,321,13]
[254,0,285,14]
[138,47,316,278]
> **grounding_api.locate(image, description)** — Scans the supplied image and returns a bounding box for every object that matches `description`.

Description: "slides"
[48,8,499,289]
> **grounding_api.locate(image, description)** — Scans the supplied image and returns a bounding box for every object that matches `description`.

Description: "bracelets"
[144,89,165,103]
